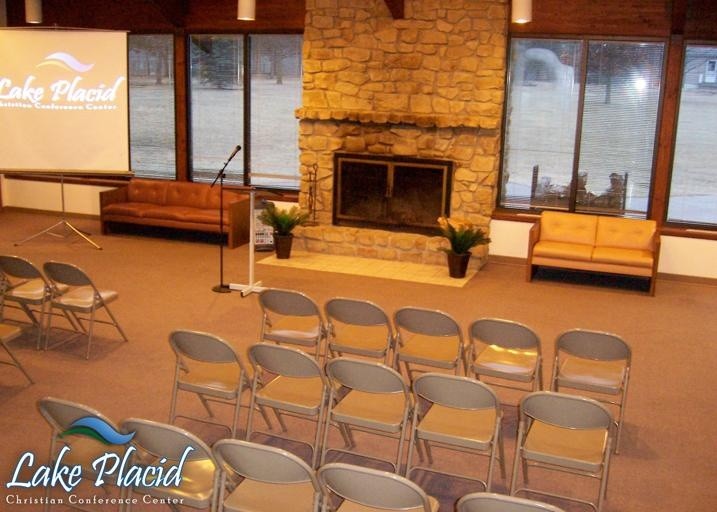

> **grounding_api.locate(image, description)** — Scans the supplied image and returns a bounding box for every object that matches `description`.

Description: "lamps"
[237,0,257,22]
[25,0,43,24]
[511,0,533,23]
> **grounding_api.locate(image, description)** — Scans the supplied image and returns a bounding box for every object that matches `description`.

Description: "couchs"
[99,177,251,249]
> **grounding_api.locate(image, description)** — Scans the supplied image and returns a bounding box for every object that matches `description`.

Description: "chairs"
[0,254,633,512]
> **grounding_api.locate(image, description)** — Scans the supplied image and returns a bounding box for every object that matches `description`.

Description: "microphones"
[227,144,244,162]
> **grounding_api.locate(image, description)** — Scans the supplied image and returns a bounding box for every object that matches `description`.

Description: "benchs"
[526,210,660,296]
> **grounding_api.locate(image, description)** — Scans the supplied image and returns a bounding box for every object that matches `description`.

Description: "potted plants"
[436,213,492,278]
[256,198,310,259]
